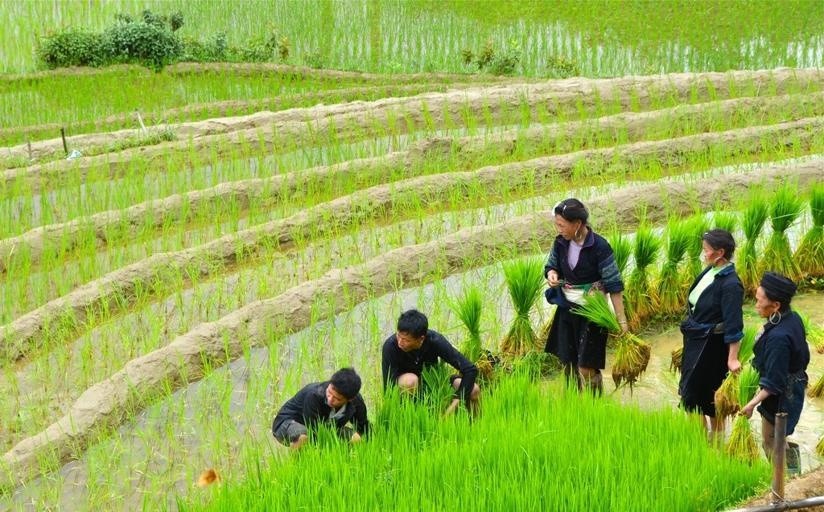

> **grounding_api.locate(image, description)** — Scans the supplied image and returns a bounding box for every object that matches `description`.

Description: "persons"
[543,197,627,399]
[381,309,479,421]
[270,366,368,453]
[737,271,810,464]
[676,229,744,433]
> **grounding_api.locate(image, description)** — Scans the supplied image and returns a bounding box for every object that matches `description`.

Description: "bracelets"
[618,320,626,324]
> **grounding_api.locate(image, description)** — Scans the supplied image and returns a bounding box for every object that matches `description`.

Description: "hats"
[760,270,798,298]
